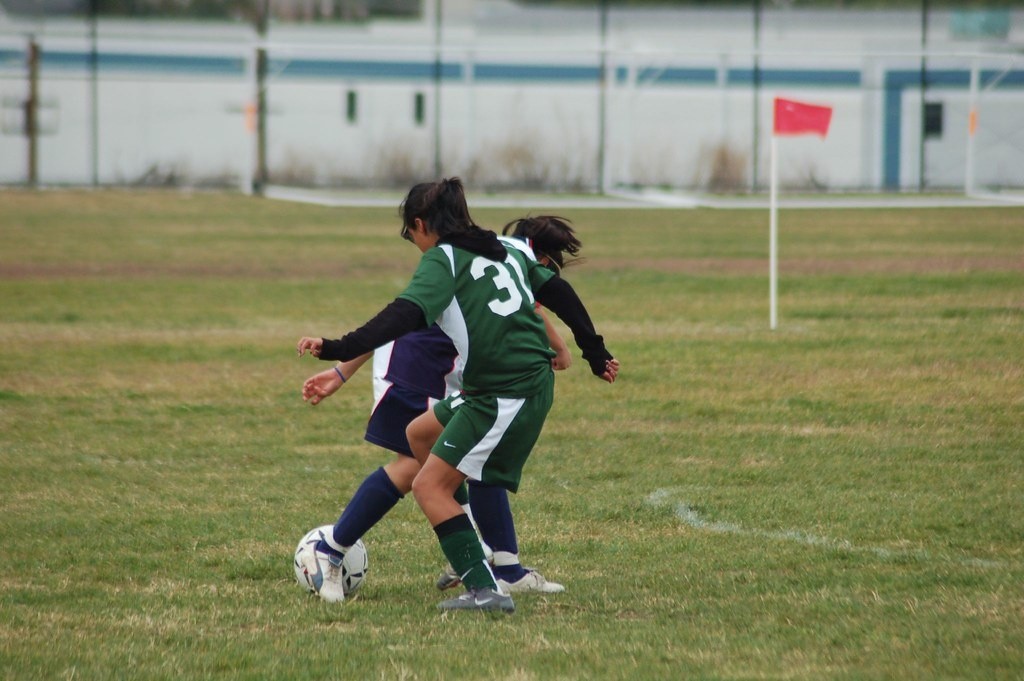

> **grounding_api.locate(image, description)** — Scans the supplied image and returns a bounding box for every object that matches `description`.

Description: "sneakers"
[496,568,564,593]
[434,587,516,615]
[437,544,492,589]
[301,530,345,604]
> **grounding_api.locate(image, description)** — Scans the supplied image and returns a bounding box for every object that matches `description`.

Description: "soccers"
[293,525,369,596]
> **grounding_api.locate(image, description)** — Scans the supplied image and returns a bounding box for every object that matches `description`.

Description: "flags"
[773,98,833,142]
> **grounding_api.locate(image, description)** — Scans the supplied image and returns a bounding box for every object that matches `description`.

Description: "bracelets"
[334,367,346,383]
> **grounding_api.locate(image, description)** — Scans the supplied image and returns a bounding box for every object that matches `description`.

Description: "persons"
[296,177,620,614]
